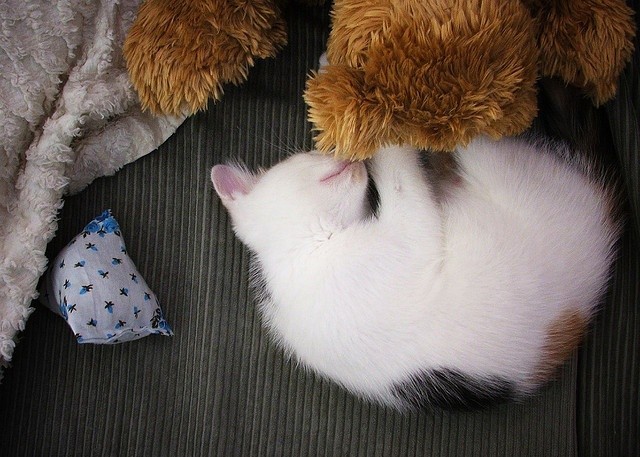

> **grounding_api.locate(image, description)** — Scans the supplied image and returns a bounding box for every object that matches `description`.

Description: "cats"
[207,52,629,421]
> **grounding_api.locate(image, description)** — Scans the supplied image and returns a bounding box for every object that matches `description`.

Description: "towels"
[0,0,195,371]
